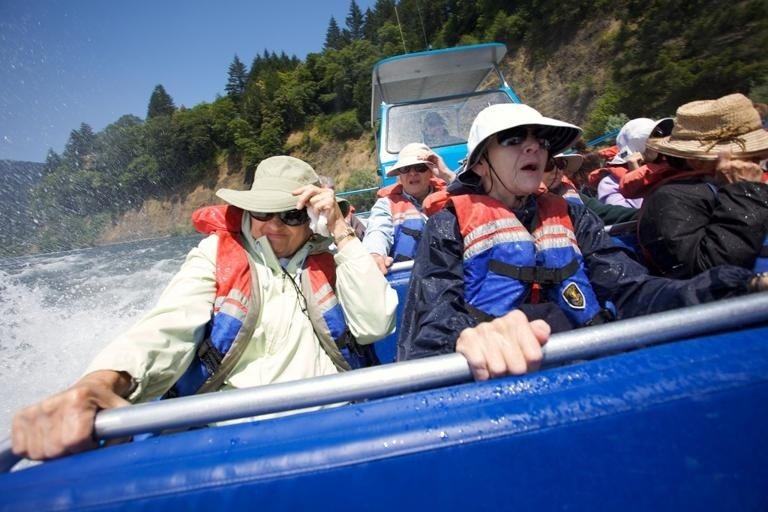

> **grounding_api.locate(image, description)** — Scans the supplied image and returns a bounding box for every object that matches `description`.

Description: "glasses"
[497,125,556,149]
[245,208,310,227]
[544,158,568,173]
[400,165,429,175]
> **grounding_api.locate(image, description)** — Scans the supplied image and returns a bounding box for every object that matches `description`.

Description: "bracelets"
[334,225,355,244]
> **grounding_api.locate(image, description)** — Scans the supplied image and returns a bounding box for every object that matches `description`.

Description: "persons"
[7,152,400,462]
[544,92,768,278]
[402,102,767,380]
[360,141,457,276]
[318,175,366,243]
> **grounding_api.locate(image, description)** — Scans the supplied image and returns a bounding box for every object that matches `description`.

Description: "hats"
[216,154,323,215]
[647,91,768,160]
[384,141,436,177]
[424,112,446,126]
[460,102,584,168]
[553,153,583,177]
[607,115,675,166]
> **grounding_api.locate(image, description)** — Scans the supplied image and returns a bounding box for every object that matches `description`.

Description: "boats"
[0,44,768,512]
